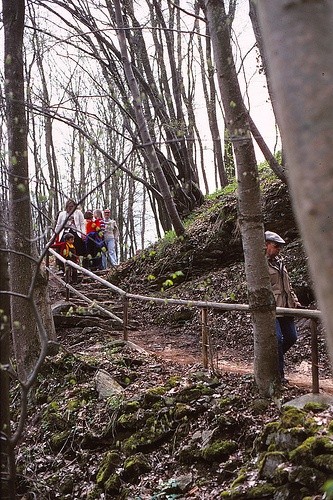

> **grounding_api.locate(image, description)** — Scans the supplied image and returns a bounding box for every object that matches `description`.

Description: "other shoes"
[57,270,64,274]
[282,378,289,384]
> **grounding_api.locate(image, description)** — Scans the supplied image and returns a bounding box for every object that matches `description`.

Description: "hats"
[265,231,286,244]
[104,209,111,213]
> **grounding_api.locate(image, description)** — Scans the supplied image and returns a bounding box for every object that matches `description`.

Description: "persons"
[265,231,301,384]
[55,200,119,276]
[50,234,79,284]
